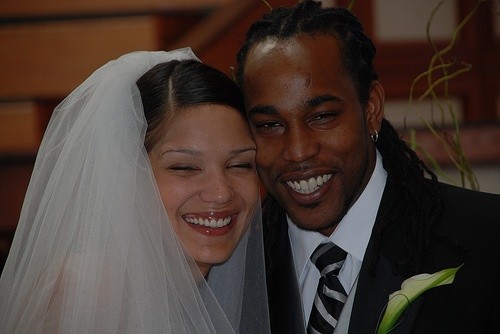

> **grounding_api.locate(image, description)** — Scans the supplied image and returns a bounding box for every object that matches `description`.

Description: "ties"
[305,243,348,334]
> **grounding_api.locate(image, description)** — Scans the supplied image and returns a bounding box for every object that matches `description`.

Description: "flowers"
[376,265,462,333]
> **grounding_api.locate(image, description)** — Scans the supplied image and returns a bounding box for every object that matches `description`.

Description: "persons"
[1,45,271,334]
[233,0,500,334]
